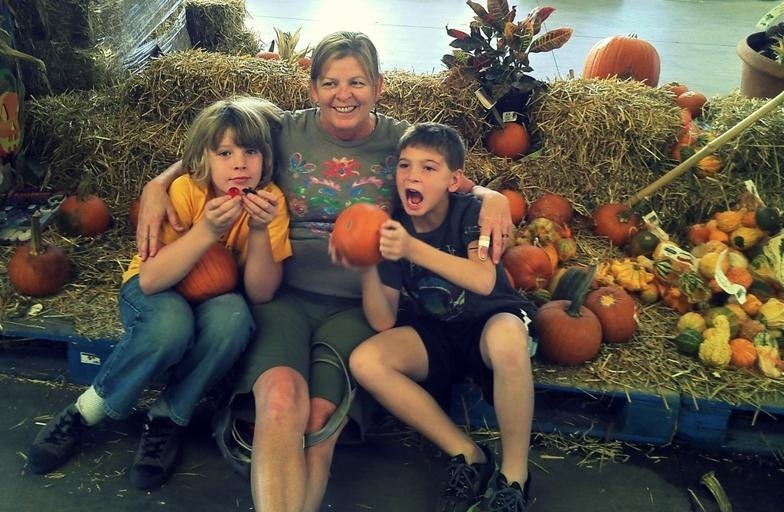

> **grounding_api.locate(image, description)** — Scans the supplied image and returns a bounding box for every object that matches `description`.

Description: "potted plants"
[441,0,572,121]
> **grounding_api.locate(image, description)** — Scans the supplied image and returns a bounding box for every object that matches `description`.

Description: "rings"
[502,233,510,238]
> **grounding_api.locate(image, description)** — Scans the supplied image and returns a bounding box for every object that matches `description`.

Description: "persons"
[29,93,292,490]
[131,29,519,512]
[347,122,536,511]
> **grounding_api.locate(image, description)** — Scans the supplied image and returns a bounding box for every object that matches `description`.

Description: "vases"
[737,32,784,98]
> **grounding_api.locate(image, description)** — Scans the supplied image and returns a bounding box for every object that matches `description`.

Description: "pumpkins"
[501,245,551,290]
[585,285,636,342]
[10,215,68,296]
[175,241,240,301]
[130,197,142,227]
[581,33,707,119]
[56,183,110,235]
[331,203,391,266]
[498,186,527,227]
[528,192,571,225]
[484,121,530,158]
[534,296,601,365]
[592,203,640,244]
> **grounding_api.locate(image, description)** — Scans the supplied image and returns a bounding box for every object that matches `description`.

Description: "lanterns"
[0,85,23,160]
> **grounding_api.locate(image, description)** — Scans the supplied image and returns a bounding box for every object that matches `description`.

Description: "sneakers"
[438,442,496,512]
[478,467,537,512]
[129,417,186,491]
[29,400,90,475]
